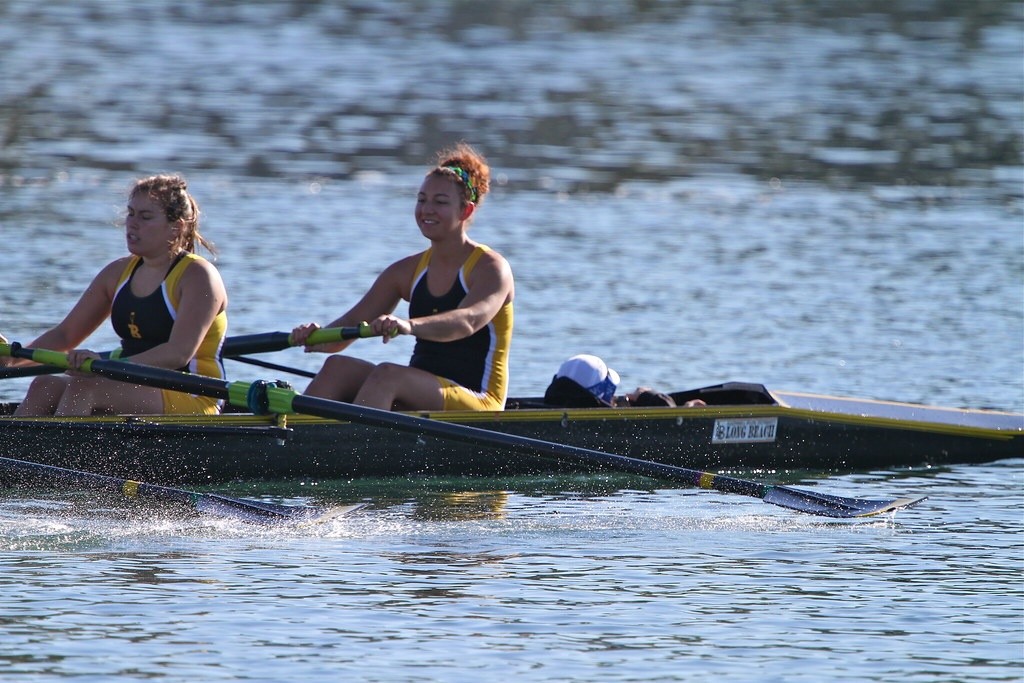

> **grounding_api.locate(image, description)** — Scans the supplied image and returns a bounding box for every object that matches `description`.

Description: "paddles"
[0,321,400,381]
[226,356,317,379]
[0,454,308,526]
[0,340,928,519]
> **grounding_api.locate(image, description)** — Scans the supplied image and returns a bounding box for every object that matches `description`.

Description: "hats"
[557,355,620,397]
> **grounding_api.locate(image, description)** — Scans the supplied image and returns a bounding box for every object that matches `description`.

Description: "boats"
[1,377,1023,477]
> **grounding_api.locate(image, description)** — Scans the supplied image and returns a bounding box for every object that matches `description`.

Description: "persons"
[302,141,515,410]
[0,175,230,417]
